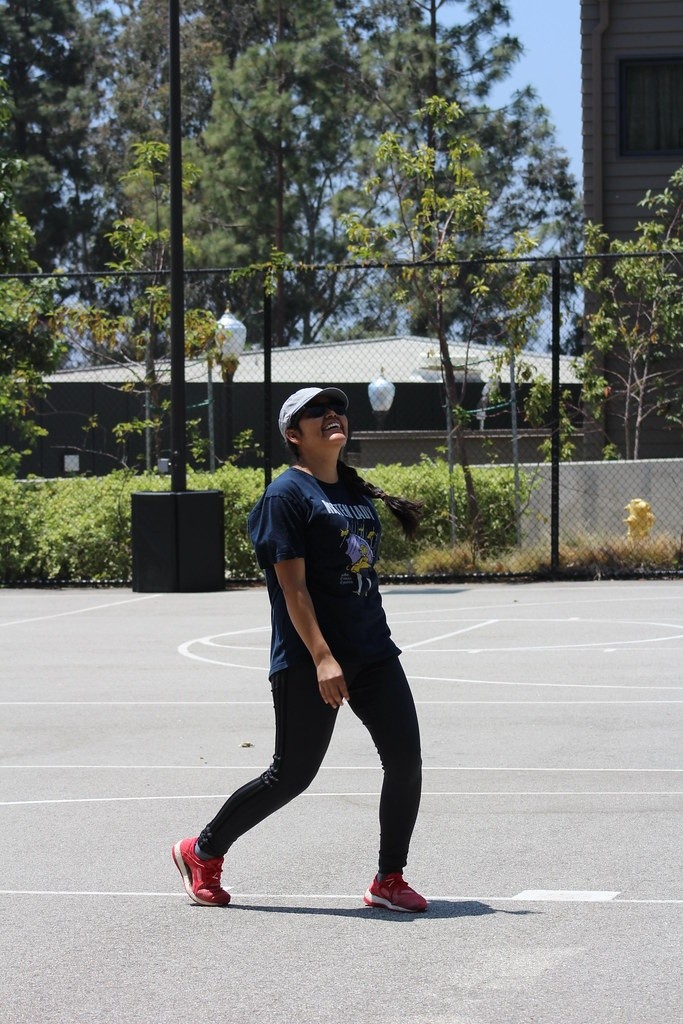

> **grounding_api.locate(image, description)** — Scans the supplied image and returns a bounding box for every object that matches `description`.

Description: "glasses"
[294,402,344,427]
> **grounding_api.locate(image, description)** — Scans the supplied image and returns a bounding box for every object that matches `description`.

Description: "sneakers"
[364,872,427,912]
[171,837,231,906]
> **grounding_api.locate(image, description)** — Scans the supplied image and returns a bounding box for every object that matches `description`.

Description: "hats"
[278,387,349,443]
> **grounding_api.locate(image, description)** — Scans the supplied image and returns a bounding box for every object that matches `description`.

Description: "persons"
[172,386,428,913]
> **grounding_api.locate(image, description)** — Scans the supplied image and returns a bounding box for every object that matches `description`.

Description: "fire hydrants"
[621,497,655,552]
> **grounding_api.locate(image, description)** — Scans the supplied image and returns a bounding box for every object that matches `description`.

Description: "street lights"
[213,301,249,466]
